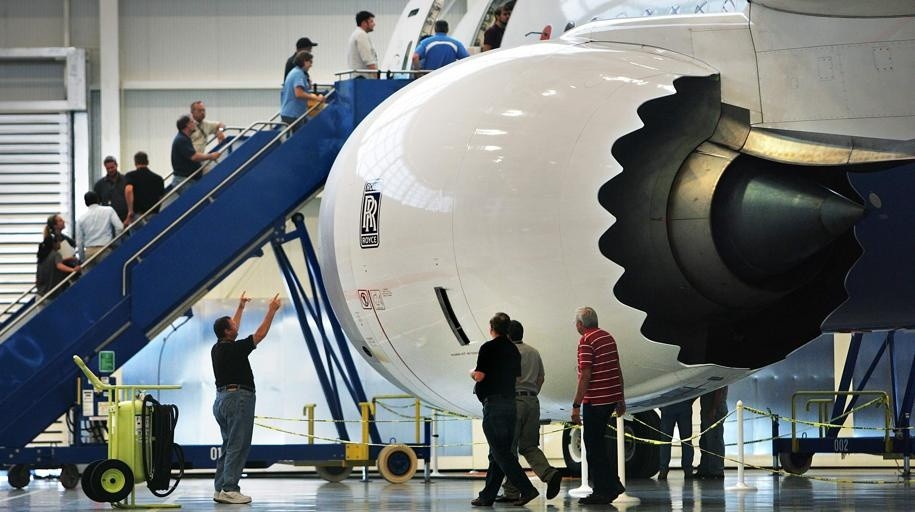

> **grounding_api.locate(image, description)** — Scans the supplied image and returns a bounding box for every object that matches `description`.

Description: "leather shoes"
[579,489,610,505]
[471,486,539,506]
[545,470,563,499]
[599,482,625,505]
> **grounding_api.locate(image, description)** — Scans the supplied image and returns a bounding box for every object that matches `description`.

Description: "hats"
[296,37,318,49]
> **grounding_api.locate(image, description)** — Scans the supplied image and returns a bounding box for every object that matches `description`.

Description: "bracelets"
[207,153,212,160]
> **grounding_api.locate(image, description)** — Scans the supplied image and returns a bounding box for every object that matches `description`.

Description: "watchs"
[573,403,580,408]
[217,128,224,132]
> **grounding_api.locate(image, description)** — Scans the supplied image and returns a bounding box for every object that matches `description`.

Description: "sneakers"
[219,489,252,504]
[214,490,221,504]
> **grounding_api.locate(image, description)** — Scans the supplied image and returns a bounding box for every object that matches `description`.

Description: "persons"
[570,306,627,504]
[124,151,164,235]
[188,101,226,171]
[495,320,561,501]
[94,156,130,249]
[75,191,123,271]
[278,1,517,134]
[659,396,697,480]
[35,236,81,300]
[695,384,728,481]
[170,115,221,195]
[211,291,281,503]
[43,215,82,285]
[470,312,539,506]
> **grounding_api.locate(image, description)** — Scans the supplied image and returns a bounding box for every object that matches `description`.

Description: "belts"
[219,384,253,392]
[515,391,536,396]
